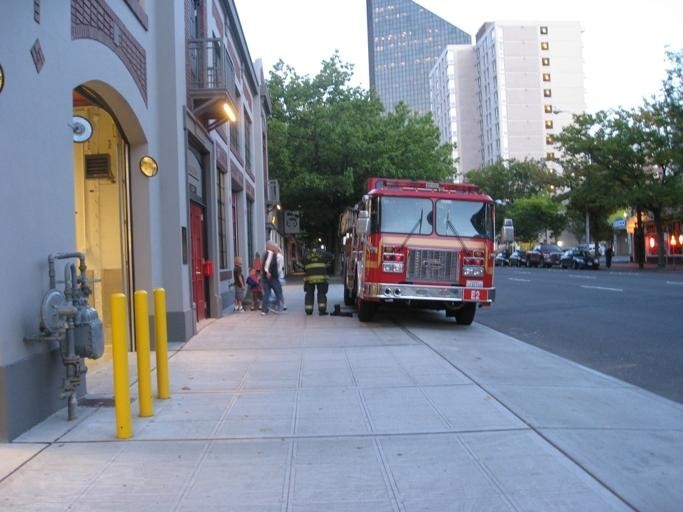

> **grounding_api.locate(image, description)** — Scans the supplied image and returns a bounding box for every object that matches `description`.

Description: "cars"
[492,242,600,270]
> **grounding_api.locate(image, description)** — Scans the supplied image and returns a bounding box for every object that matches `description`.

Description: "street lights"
[552,108,590,244]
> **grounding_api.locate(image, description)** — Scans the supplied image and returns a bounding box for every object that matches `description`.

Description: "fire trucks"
[337,176,497,326]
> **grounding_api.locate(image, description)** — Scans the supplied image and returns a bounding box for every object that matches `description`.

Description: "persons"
[235,239,286,315]
[303,240,335,315]
[605,245,614,267]
